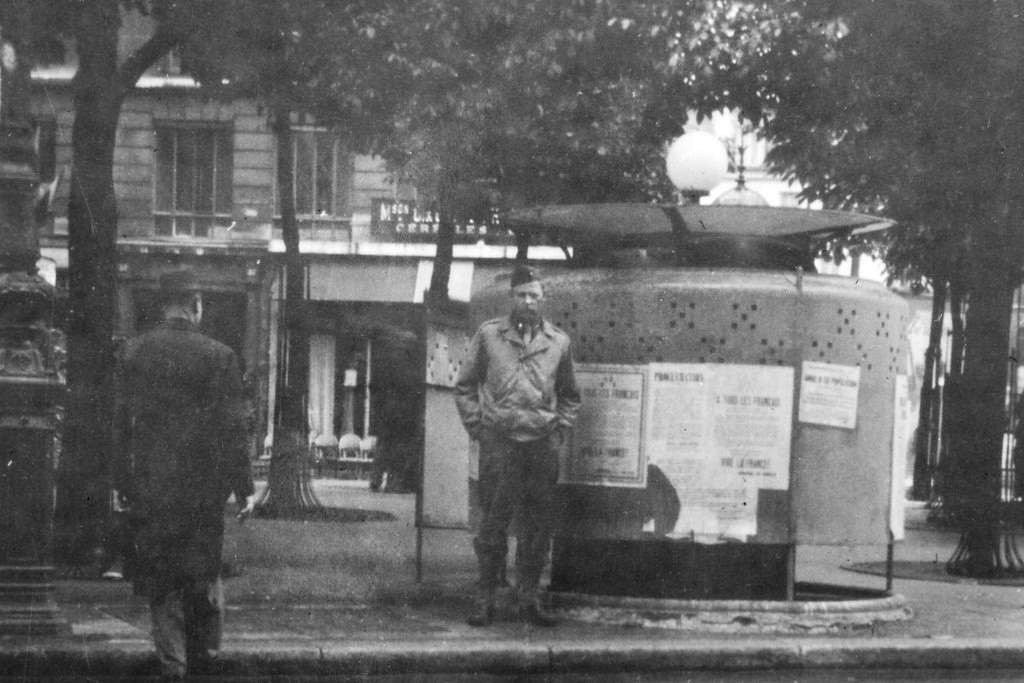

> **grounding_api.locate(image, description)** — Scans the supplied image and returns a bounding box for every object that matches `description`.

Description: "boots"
[468,537,509,627]
[517,562,559,627]
[150,589,190,683]
[185,573,226,669]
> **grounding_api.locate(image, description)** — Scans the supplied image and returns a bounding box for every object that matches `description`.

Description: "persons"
[106,265,258,683]
[454,258,586,627]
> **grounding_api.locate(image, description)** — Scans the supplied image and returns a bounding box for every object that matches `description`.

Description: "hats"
[511,262,539,286]
[160,267,199,294]
